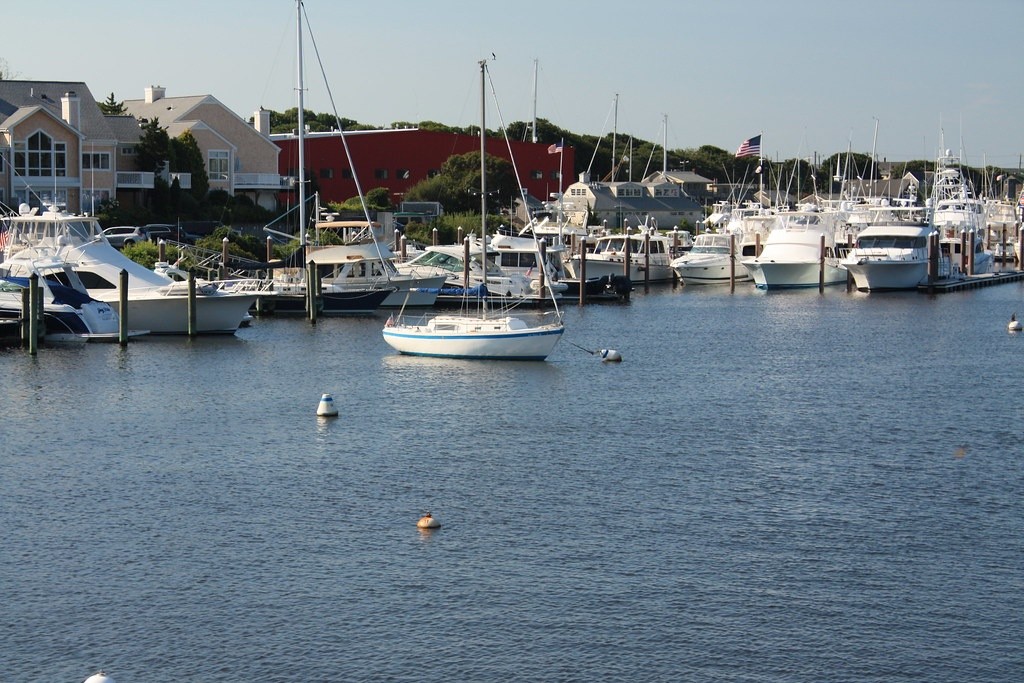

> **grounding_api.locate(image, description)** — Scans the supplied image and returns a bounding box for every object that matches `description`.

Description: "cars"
[93,226,148,250]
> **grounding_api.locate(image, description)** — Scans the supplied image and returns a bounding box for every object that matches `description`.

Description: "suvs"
[145,224,200,245]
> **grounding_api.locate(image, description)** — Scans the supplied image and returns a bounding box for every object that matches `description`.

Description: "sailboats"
[380,56,567,361]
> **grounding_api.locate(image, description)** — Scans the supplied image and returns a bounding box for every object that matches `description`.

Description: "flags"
[735,135,760,157]
[0,215,9,251]
[548,142,561,154]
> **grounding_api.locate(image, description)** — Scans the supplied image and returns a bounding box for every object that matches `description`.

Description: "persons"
[57,230,72,245]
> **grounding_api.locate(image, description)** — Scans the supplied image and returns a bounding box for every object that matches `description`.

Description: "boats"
[0,0,1024,344]
